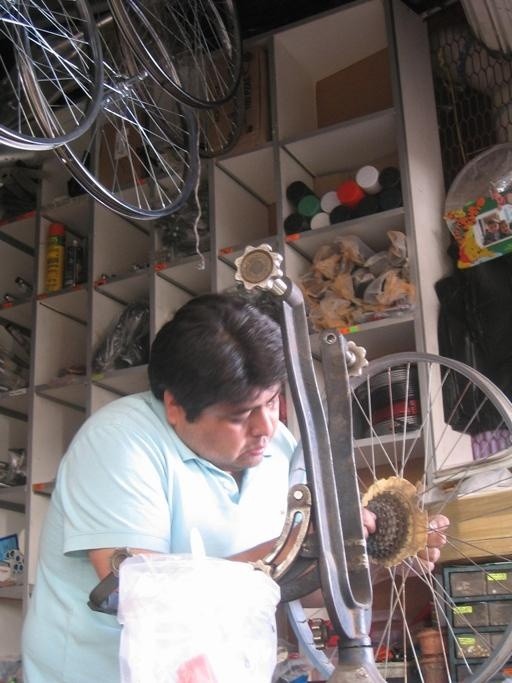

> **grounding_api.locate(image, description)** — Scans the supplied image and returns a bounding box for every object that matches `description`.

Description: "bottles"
[46,222,65,292]
[285,162,402,235]
[64,238,87,286]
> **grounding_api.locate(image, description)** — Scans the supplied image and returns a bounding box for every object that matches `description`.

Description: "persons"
[484,218,512,245]
[22,295,451,683]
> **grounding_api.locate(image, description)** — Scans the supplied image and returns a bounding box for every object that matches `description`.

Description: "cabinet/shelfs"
[443,563,512,683]
[0,0,473,683]
